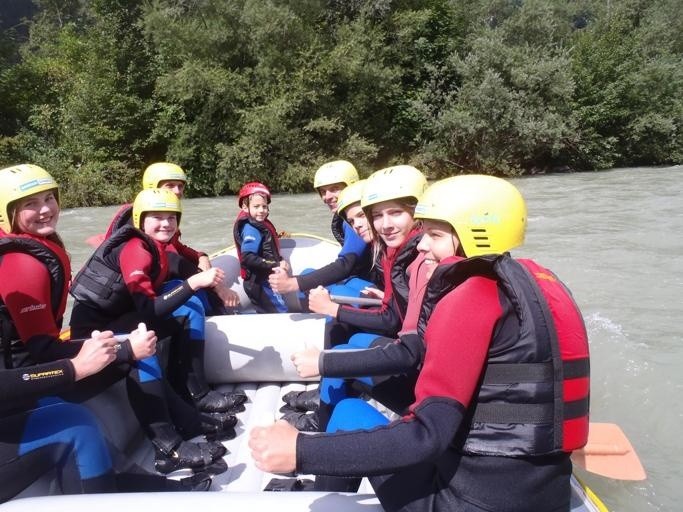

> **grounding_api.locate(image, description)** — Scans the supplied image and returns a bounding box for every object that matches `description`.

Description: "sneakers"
[194,389,246,411]
[284,384,325,408]
[181,473,211,492]
[153,437,226,475]
[282,410,325,430]
[177,411,236,440]
[269,478,314,491]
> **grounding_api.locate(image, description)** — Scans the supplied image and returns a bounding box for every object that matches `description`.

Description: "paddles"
[299,413,649,481]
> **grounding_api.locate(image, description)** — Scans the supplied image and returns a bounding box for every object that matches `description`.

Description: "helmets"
[361,165,429,230]
[238,184,271,208]
[132,190,182,230]
[314,160,360,199]
[0,164,60,233]
[143,163,186,190]
[414,174,526,259]
[337,179,369,223]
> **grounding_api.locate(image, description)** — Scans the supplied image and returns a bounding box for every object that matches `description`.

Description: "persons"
[0,164,237,490]
[102,160,240,316]
[308,177,383,413]
[0,330,211,506]
[247,172,590,512]
[280,164,432,431]
[234,181,291,315]
[68,189,248,413]
[267,159,375,323]
